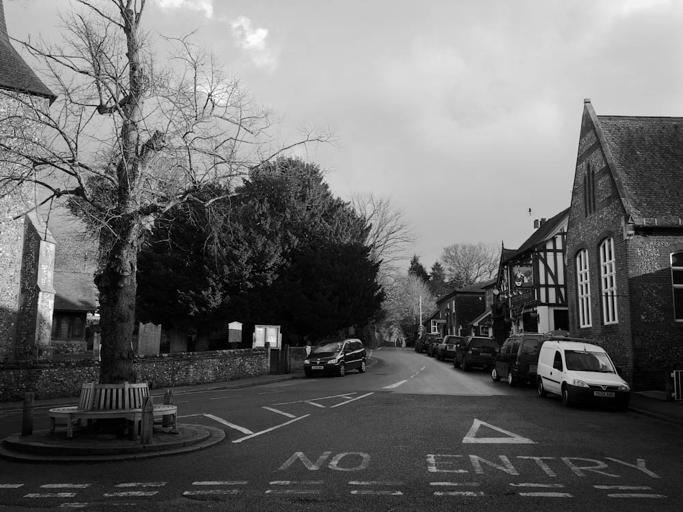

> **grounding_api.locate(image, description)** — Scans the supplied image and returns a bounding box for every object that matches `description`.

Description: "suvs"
[452,335,499,372]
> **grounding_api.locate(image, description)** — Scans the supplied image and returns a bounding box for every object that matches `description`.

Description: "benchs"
[47,382,178,440]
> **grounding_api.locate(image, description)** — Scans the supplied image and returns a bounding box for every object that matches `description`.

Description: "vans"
[304,338,367,378]
[491,331,548,388]
[536,339,632,410]
